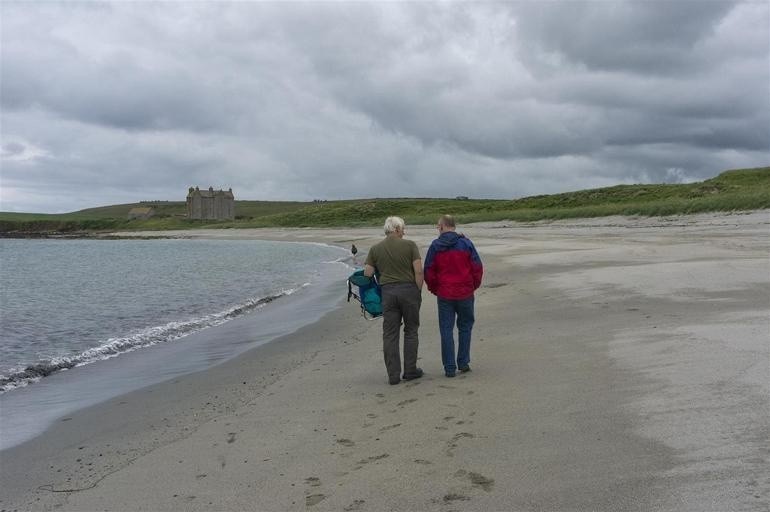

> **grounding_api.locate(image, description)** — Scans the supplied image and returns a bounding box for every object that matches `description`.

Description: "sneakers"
[390,368,423,385]
[446,365,469,377]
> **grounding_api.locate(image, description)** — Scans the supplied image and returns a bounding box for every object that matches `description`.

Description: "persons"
[363,216,425,385]
[423,214,484,378]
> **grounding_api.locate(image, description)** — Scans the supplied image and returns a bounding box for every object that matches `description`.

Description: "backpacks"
[346,267,383,321]
